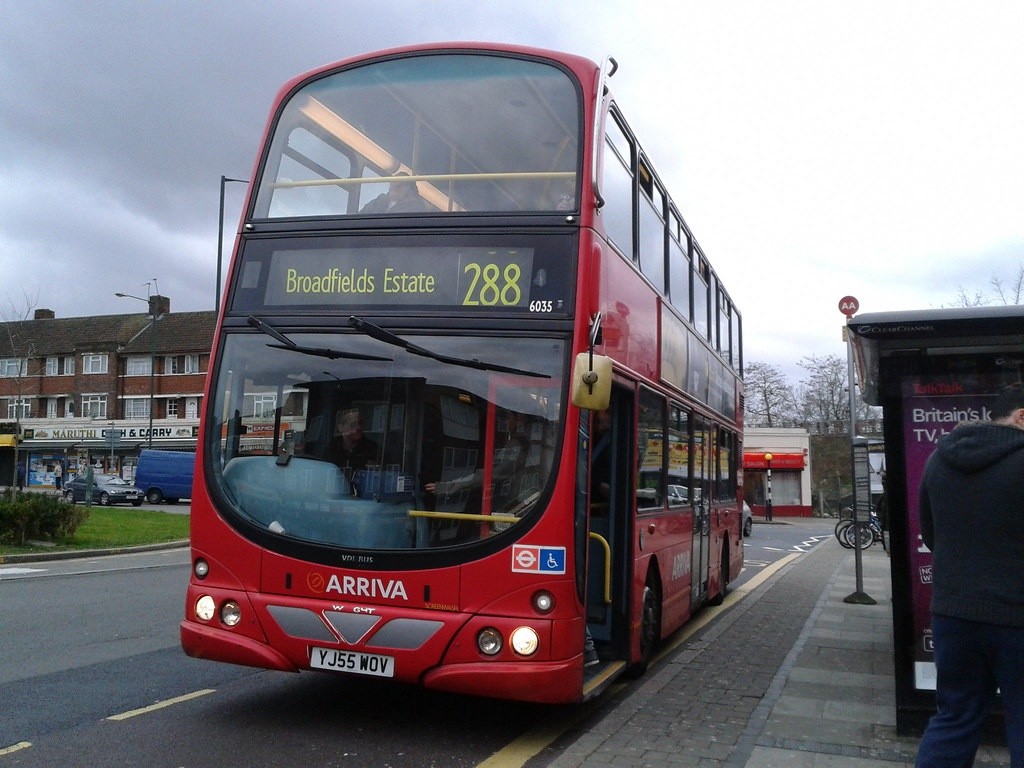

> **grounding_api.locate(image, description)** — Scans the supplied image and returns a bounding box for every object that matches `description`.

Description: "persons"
[292,399,389,497]
[15,460,26,491]
[426,400,599,667]
[915,381,1024,768]
[54,464,63,490]
[360,174,427,212]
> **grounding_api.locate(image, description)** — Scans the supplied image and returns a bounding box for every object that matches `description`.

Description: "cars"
[62,473,145,506]
[635,483,734,537]
[741,499,752,537]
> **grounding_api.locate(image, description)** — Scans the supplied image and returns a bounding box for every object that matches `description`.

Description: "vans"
[133,449,196,506]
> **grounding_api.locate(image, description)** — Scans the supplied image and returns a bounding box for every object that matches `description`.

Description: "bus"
[179,41,745,707]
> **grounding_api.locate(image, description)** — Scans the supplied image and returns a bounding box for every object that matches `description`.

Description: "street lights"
[115,293,159,450]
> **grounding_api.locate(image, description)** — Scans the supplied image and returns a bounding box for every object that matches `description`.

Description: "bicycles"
[834,503,885,550]
